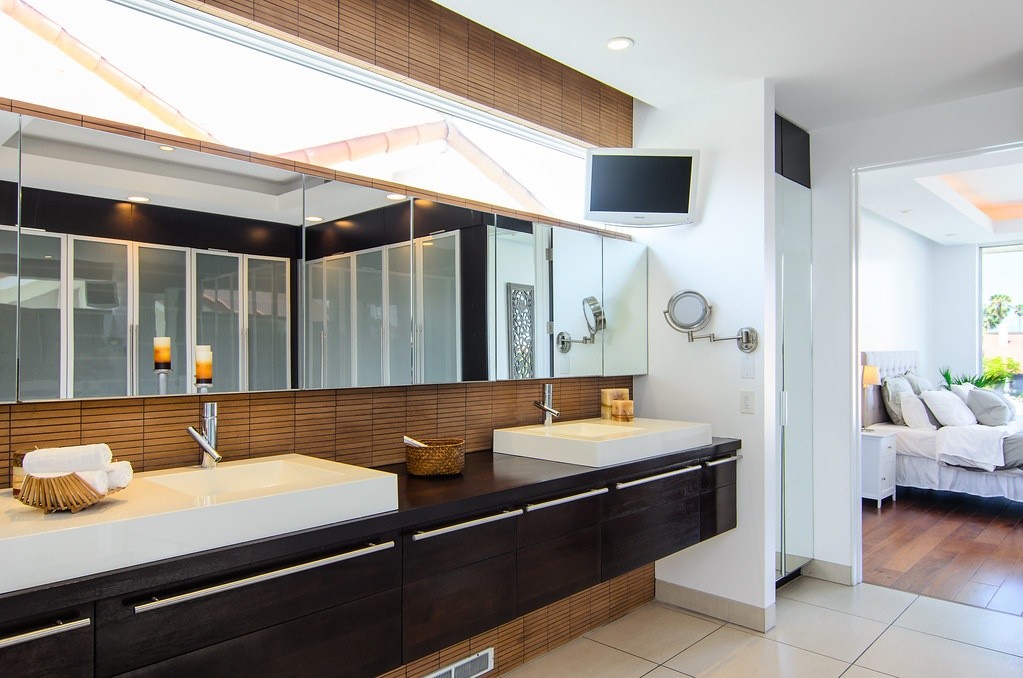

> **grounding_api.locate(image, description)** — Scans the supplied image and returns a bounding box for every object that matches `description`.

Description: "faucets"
[186,401,222,468]
[533,383,560,426]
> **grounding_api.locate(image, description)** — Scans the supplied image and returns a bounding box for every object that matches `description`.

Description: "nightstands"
[860,429,897,509]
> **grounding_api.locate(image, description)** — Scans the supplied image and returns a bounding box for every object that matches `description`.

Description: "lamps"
[194,344,212,395]
[860,365,882,434]
[154,336,172,396]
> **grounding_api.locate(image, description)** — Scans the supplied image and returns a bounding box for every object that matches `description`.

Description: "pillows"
[918,390,979,426]
[904,370,939,395]
[966,389,1010,426]
[880,373,915,426]
[949,382,978,406]
[973,386,1016,422]
[898,391,942,431]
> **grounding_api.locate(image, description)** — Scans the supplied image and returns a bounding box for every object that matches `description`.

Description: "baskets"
[407,438,465,476]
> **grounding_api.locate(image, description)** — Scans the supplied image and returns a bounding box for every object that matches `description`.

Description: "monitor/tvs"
[84,280,120,310]
[583,148,699,228]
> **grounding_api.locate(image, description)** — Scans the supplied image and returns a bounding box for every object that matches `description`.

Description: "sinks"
[493,417,712,468]
[144,460,345,497]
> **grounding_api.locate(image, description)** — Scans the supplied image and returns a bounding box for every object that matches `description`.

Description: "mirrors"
[662,290,758,355]
[555,296,607,355]
[0,108,648,406]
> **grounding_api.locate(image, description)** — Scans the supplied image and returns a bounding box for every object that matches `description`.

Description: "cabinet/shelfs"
[0,437,743,678]
[773,112,814,583]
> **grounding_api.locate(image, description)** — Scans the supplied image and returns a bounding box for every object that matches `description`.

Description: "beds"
[860,349,1023,503]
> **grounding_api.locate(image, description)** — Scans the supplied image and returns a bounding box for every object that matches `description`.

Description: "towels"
[23,442,114,474]
[106,460,134,489]
[21,473,109,503]
